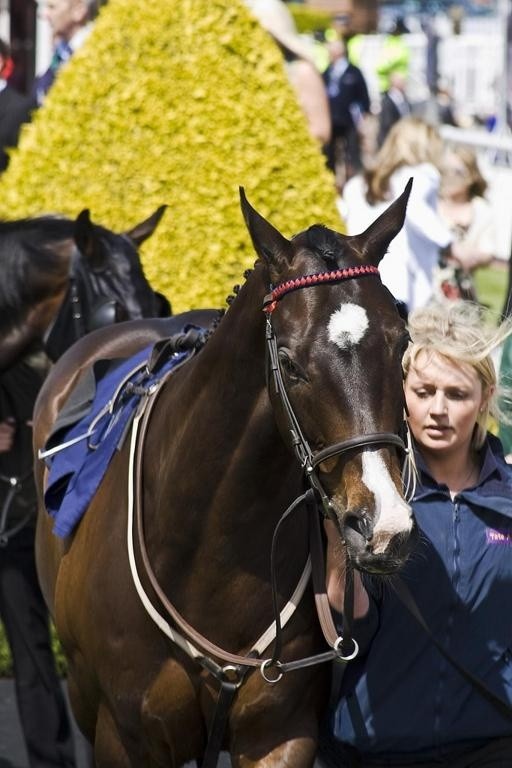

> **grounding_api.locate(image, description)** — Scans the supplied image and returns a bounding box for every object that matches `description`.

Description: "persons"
[326,301,512,768]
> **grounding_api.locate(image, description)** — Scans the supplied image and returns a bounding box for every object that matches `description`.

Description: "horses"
[1,202,170,765]
[29,173,425,768]
